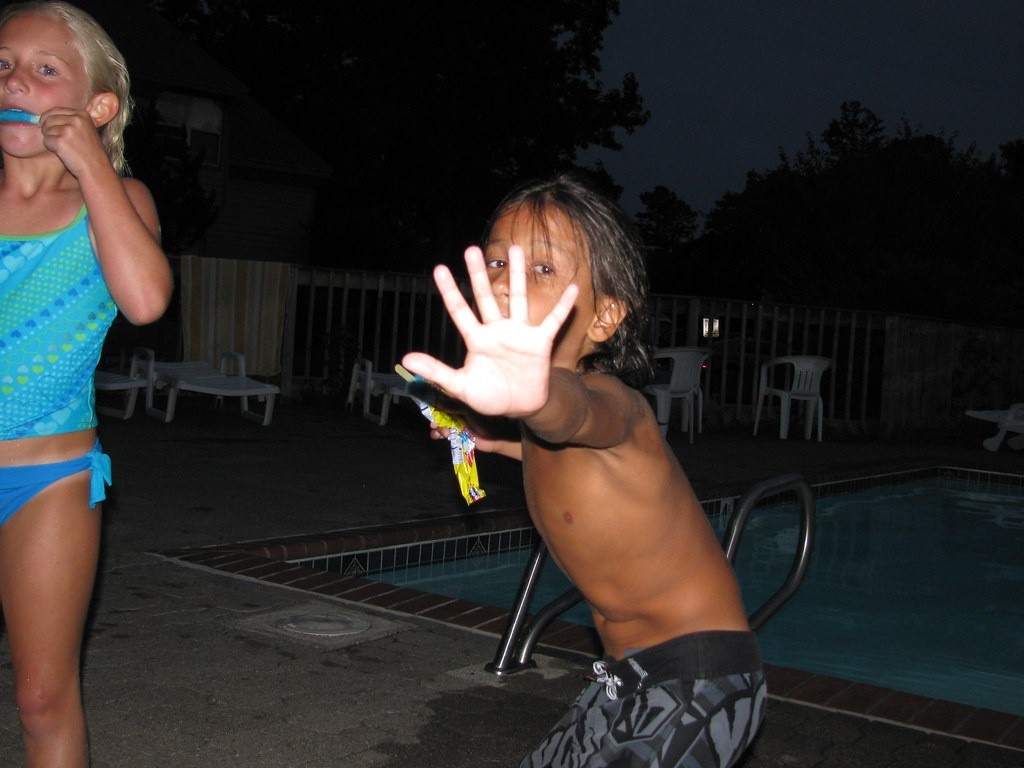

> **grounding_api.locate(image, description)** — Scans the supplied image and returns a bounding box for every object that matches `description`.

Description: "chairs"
[646,347,713,446]
[95,368,148,419]
[116,347,281,426]
[344,360,409,424]
[965,403,1024,452]
[754,355,833,442]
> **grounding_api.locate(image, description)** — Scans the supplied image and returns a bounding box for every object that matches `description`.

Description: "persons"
[401,172,769,768]
[0,0,172,768]
